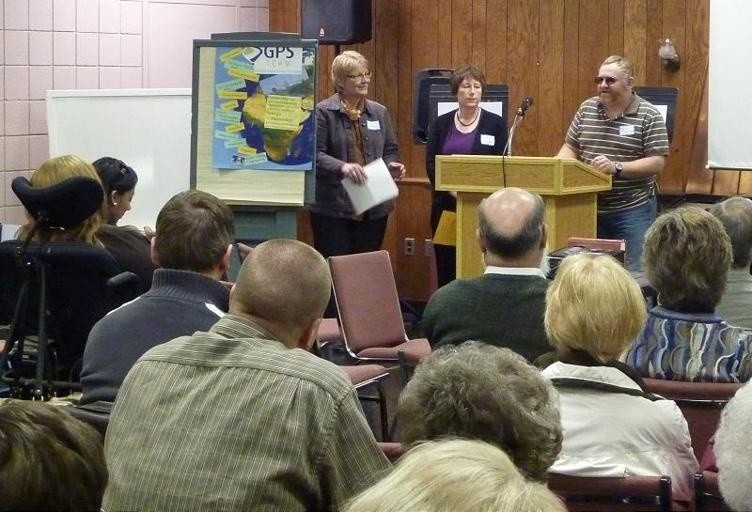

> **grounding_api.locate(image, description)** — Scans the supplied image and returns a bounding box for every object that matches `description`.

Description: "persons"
[80,188,235,409]
[100,236,393,512]
[309,50,406,326]
[556,56,671,306]
[1,397,109,512]
[332,186,752,511]
[425,63,509,286]
[15,155,154,253]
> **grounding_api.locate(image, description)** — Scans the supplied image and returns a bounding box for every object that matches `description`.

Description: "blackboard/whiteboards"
[45,87,193,235]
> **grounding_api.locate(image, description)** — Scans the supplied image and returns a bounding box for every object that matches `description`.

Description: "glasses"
[347,71,372,81]
[594,76,629,85]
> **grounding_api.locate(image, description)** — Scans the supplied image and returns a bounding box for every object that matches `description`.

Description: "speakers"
[300,0,371,45]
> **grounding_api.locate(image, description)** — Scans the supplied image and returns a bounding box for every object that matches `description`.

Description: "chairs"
[2,238,143,441]
[540,466,732,511]
[311,249,431,462]
[640,376,746,465]
[216,242,252,290]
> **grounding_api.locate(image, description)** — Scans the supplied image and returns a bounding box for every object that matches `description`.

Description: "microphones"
[517,96,532,115]
[502,114,526,155]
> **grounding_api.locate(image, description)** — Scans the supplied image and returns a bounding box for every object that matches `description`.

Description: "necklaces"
[457,108,479,127]
